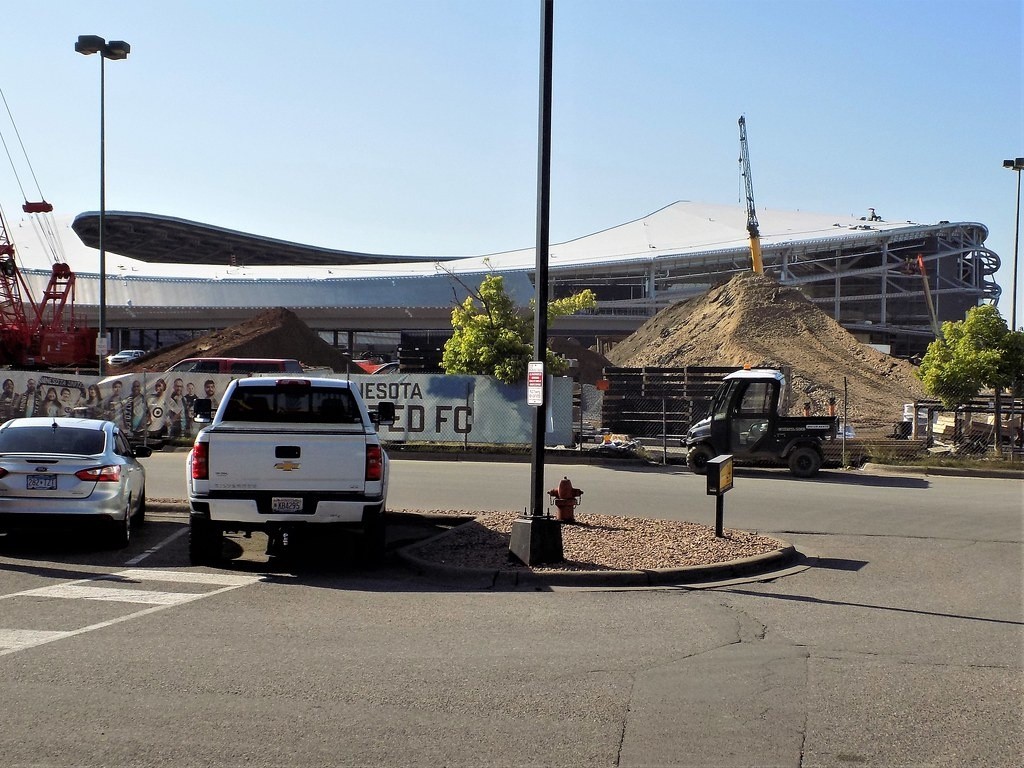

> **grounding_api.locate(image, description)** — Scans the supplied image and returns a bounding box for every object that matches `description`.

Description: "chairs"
[316,398,345,412]
[242,396,269,413]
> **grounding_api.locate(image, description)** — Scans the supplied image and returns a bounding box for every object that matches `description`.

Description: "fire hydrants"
[547,474,583,524]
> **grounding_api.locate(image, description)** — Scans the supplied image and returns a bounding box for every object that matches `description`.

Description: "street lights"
[142,363,155,446]
[1001,156,1024,332]
[73,33,130,376]
[341,351,353,414]
[566,336,583,453]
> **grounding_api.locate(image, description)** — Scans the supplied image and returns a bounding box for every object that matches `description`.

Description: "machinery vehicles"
[732,114,765,276]
[900,255,995,433]
[0,88,99,376]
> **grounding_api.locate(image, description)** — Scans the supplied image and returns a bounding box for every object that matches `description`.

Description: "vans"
[162,357,306,374]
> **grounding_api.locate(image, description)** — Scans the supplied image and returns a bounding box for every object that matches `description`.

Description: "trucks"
[679,363,841,478]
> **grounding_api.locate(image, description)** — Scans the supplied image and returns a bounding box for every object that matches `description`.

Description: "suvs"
[184,374,396,567]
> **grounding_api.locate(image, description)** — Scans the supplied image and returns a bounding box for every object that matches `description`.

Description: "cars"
[110,348,146,366]
[0,414,152,550]
